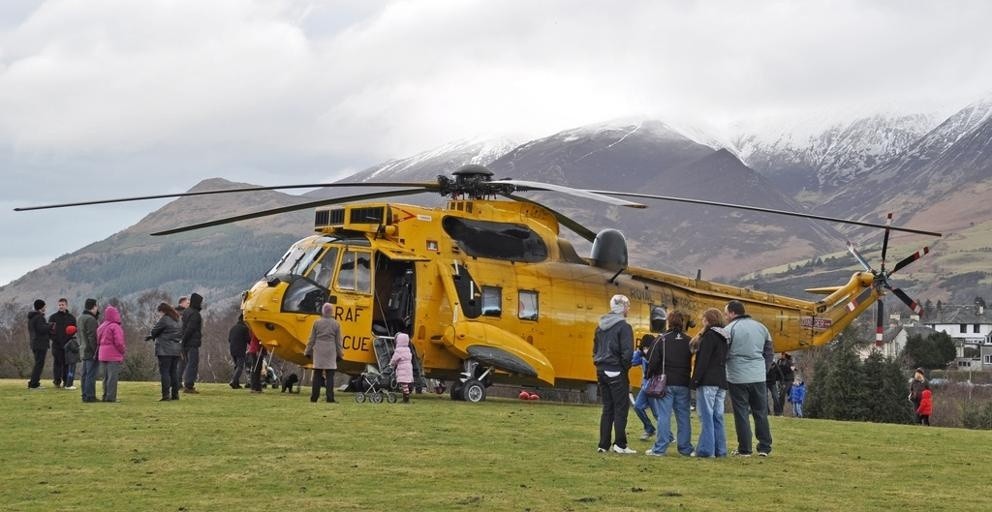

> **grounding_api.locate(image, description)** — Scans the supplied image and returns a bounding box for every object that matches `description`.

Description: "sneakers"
[597,432,667,456]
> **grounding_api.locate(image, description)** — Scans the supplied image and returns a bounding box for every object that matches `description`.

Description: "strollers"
[244,357,282,389]
[356,363,400,404]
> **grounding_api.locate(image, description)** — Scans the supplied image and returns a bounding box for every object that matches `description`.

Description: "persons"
[776,352,794,413]
[764,359,785,418]
[591,293,638,455]
[629,298,775,458]
[787,373,806,419]
[391,332,417,401]
[26,292,279,394]
[305,303,346,403]
[914,385,932,426]
[907,368,929,424]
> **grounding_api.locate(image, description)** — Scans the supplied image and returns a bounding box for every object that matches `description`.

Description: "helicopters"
[8,163,944,403]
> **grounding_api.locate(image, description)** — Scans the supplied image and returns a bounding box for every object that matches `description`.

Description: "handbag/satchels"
[645,374,667,398]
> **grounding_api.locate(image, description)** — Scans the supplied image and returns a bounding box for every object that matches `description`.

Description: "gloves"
[144,336,151,341]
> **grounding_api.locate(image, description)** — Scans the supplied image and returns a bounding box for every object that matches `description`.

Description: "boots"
[399,393,410,404]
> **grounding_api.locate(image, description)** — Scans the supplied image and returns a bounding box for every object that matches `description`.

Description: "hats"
[66,326,77,336]
[34,300,45,310]
